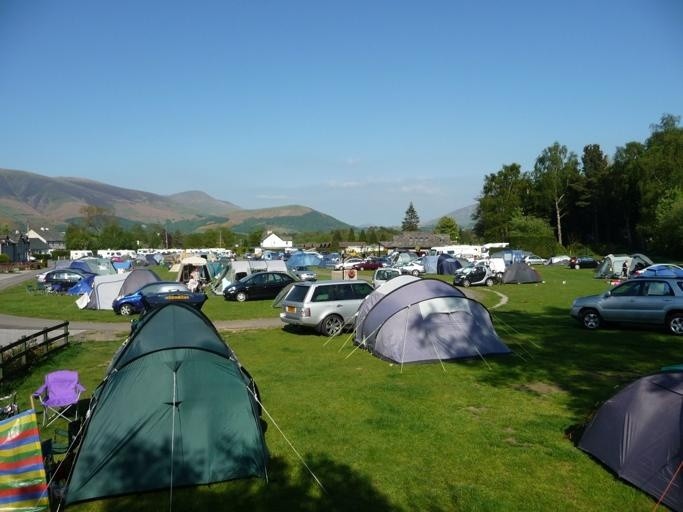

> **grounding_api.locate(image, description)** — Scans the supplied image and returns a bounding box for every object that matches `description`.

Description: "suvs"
[36,267,95,295]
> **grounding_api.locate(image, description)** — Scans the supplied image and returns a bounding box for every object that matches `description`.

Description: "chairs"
[32,369,86,429]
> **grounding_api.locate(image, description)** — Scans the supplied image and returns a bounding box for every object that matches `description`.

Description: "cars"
[318,244,600,286]
[109,281,208,312]
[566,275,680,338]
[221,271,298,304]
[278,279,375,336]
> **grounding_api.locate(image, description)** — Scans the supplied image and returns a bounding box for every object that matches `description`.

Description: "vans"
[68,248,233,260]
